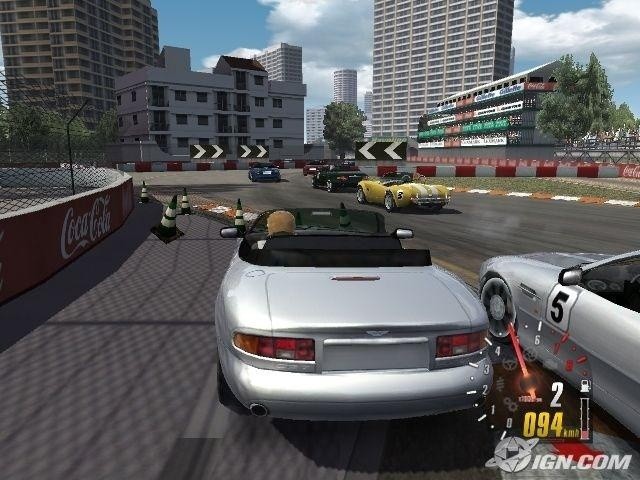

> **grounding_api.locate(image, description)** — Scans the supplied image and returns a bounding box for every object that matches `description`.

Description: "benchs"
[260,232,403,250]
